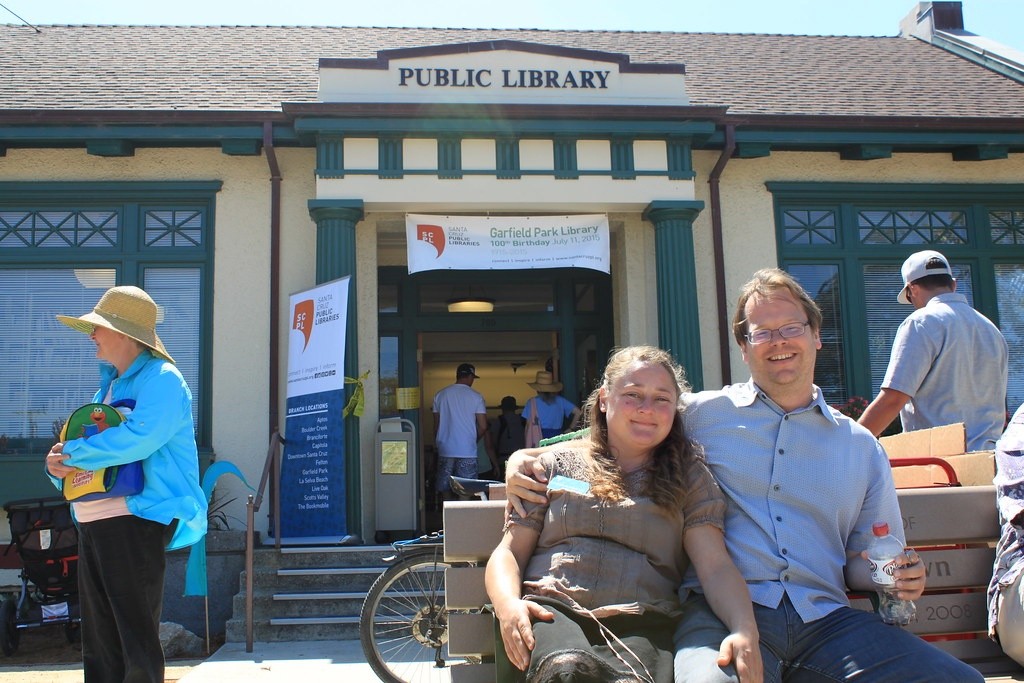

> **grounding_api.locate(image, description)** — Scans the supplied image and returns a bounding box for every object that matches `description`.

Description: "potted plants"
[159,488,261,642]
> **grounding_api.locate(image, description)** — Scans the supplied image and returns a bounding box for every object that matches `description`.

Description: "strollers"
[0,496,82,657]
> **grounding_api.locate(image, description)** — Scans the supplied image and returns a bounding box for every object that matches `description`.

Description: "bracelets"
[568,426,575,431]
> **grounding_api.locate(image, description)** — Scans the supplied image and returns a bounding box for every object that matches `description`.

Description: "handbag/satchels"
[524,397,543,448]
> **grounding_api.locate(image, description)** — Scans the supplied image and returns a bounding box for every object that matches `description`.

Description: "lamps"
[447,284,495,312]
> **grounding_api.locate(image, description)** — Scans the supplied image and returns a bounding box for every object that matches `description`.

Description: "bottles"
[865,522,916,626]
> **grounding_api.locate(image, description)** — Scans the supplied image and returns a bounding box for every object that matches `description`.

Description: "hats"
[55,285,176,364]
[456,363,480,379]
[896,250,953,305]
[527,371,564,393]
[497,395,520,411]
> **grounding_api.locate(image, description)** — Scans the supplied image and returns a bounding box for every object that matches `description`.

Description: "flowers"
[830,396,870,422]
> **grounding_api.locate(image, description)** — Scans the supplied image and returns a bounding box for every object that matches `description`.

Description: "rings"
[905,552,912,564]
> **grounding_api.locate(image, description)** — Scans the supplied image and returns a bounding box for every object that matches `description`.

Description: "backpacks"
[58,398,145,503]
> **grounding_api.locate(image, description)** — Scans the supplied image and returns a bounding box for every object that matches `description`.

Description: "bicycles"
[357,475,503,683]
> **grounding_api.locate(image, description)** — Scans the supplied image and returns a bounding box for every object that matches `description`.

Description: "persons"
[43,285,209,683]
[432,365,488,500]
[476,409,501,481]
[485,344,764,683]
[521,370,581,448]
[504,266,987,683]
[490,396,527,485]
[986,401,1024,666]
[856,251,1009,454]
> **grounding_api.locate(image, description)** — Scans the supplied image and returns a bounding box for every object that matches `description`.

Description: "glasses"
[743,321,811,345]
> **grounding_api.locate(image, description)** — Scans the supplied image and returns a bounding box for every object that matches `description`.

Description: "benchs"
[443,484,1024,683]
